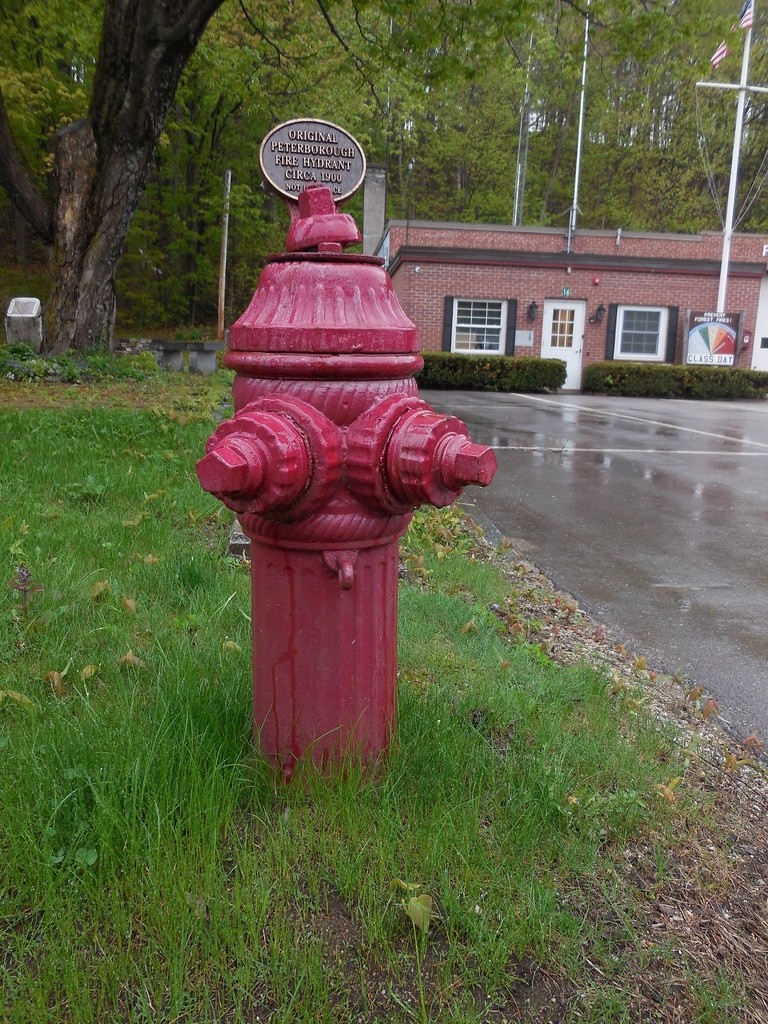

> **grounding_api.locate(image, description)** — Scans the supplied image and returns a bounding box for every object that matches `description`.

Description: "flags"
[709,0,754,71]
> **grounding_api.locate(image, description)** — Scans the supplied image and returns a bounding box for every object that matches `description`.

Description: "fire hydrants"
[195,180,498,792]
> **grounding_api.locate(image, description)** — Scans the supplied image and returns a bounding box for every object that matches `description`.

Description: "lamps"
[589,304,605,323]
[529,300,538,320]
